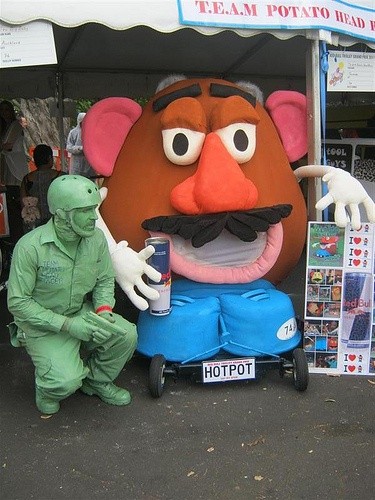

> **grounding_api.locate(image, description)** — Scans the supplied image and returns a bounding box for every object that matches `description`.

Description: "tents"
[0,0,375,223]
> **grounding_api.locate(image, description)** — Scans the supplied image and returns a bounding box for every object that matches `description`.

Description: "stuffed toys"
[82,73,375,363]
[21,197,40,223]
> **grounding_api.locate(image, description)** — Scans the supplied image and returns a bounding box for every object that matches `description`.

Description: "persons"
[19,144,68,234]
[67,112,96,182]
[8,174,137,414]
[0,100,28,244]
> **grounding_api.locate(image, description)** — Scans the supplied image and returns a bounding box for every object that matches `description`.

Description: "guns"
[81,310,127,337]
